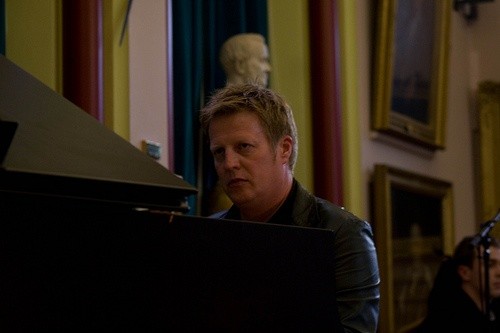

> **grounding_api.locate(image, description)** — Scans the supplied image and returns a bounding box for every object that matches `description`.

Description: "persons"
[220,34,269,87]
[424,235,500,333]
[205,83,381,333]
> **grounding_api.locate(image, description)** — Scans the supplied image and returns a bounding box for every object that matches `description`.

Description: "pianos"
[0,53,336,333]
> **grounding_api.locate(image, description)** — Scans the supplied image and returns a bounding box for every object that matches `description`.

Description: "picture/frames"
[372,0,452,151]
[373,163,456,333]
